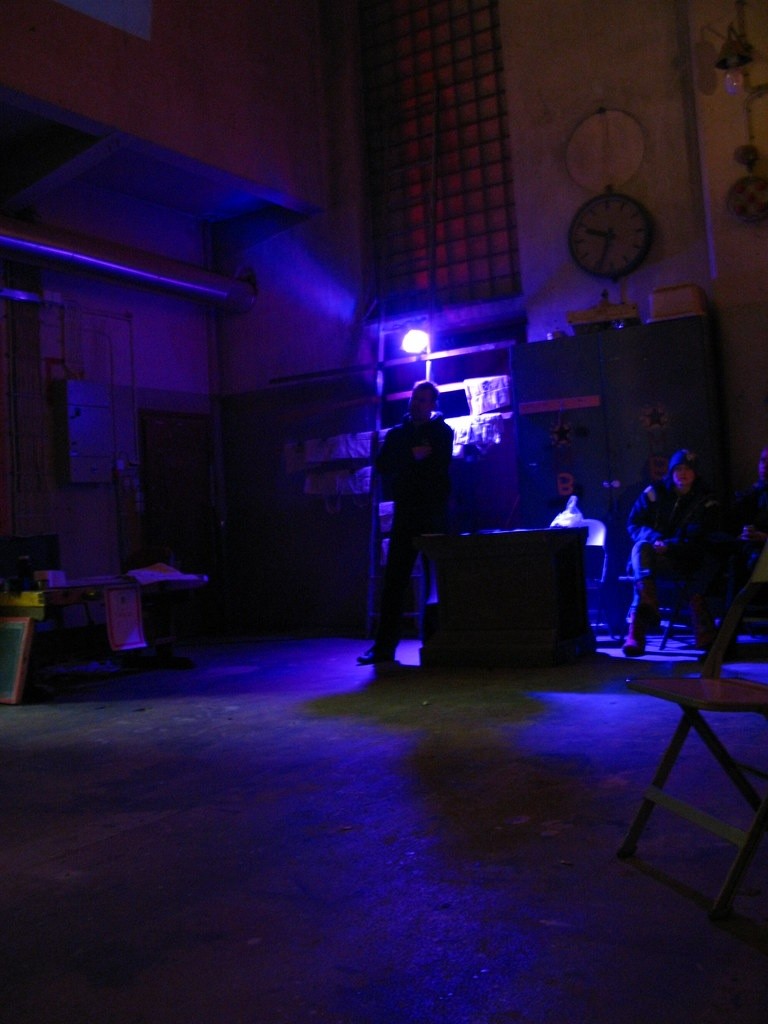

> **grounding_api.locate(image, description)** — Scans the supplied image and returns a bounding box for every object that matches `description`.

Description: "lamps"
[715,23,754,97]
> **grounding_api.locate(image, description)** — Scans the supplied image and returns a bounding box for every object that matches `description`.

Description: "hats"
[668,450,698,475]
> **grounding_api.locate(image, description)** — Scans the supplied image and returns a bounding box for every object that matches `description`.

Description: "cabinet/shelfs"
[502,318,729,646]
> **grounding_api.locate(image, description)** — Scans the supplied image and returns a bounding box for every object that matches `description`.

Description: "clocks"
[566,193,654,282]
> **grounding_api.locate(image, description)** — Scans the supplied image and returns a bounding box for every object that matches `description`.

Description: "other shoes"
[357,650,395,664]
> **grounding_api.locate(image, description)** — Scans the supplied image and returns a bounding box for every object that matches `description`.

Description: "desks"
[411,528,597,675]
[0,575,209,699]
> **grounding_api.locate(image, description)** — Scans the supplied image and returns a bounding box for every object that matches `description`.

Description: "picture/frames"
[0,616,36,705]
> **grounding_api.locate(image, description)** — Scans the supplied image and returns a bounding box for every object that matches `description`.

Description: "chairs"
[614,542,768,922]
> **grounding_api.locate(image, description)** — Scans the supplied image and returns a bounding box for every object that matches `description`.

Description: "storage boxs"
[568,302,642,335]
[650,284,712,320]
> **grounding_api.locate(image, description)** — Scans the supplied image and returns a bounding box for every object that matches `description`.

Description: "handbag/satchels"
[549,496,583,528]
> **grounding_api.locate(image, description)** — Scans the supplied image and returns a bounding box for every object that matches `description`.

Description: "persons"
[623,447,768,656]
[357,383,454,673]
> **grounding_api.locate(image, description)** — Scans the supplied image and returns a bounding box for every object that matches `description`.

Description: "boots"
[690,593,719,649]
[623,611,645,656]
